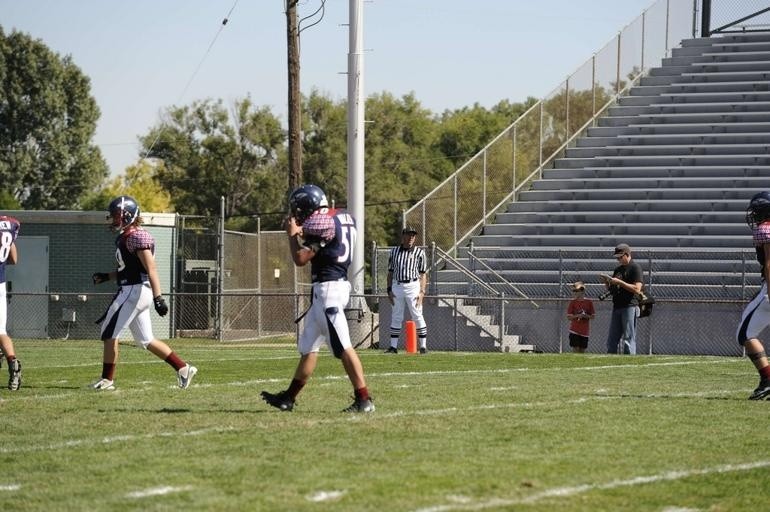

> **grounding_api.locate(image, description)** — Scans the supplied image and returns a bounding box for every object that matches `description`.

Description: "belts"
[400,278,418,283]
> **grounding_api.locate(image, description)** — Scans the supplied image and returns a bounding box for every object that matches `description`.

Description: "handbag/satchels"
[635,288,655,318]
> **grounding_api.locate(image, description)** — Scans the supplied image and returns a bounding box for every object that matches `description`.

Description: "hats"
[401,226,418,237]
[613,244,631,258]
[572,281,585,292]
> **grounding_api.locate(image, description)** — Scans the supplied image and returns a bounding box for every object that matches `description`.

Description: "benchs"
[427,36,769,298]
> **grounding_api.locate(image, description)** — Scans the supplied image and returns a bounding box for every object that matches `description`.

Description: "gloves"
[93,272,109,285]
[154,294,167,317]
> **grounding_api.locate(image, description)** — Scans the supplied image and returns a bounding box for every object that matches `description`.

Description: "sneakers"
[342,397,375,413]
[419,347,427,353]
[92,378,115,390]
[7,360,22,391]
[260,390,294,412]
[749,381,770,401]
[176,363,198,389]
[384,346,397,354]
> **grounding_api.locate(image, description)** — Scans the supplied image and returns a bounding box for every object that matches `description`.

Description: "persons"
[736,191,770,401]
[0,215,23,391]
[89,196,198,391]
[567,282,596,353]
[260,185,375,415]
[600,243,643,355]
[384,228,428,354]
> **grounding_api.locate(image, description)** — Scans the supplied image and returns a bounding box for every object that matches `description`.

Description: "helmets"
[289,184,329,225]
[745,189,770,229]
[106,196,138,231]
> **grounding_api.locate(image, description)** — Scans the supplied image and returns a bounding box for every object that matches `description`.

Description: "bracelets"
[387,287,391,294]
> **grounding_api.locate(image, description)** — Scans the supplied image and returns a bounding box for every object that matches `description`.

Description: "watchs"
[420,290,425,294]
